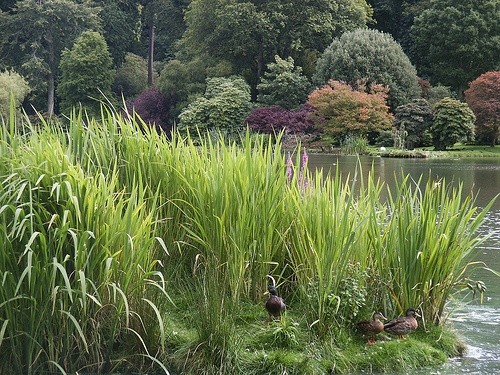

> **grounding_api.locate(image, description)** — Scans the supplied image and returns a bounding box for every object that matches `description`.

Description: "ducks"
[263,286,286,319]
[356,311,388,344]
[384,308,421,341]
